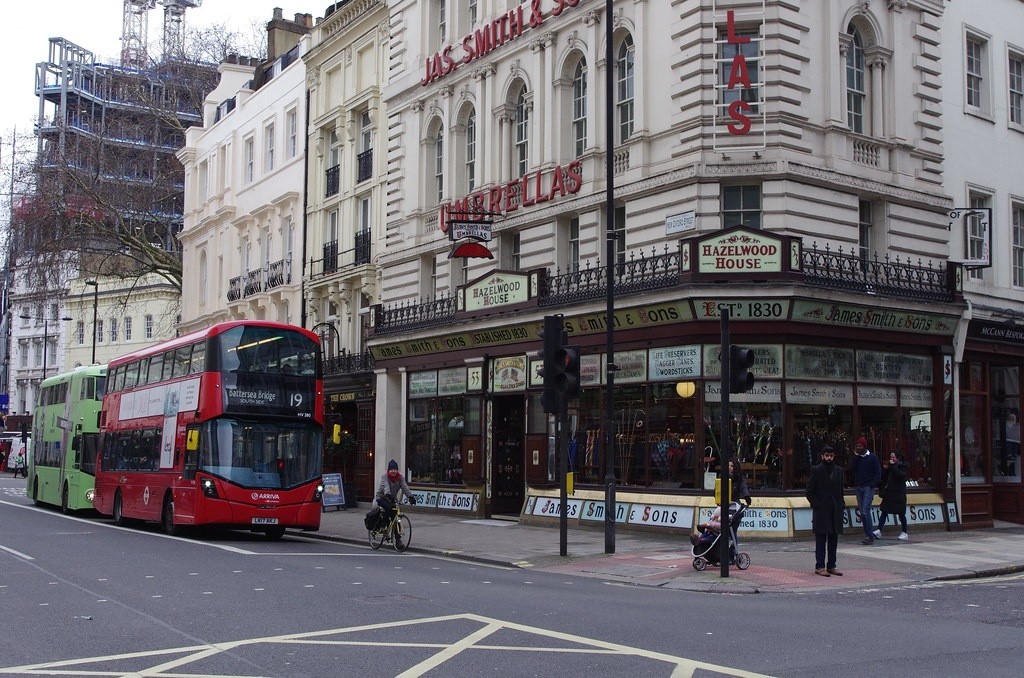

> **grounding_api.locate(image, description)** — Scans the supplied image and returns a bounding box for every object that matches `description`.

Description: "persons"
[376,459,416,550]
[0,453,5,464]
[805,447,845,577]
[170,395,178,414]
[849,437,882,544]
[713,460,751,564]
[13,453,26,478]
[872,449,909,540]
[776,449,795,489]
[690,505,721,546]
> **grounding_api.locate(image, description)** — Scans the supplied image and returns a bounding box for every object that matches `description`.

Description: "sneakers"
[873,529,881,539]
[898,532,908,540]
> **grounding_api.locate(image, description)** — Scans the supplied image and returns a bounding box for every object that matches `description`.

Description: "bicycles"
[368,499,412,553]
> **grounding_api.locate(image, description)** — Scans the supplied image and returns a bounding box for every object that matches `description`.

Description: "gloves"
[409,497,416,505]
[389,500,396,509]
[744,495,751,505]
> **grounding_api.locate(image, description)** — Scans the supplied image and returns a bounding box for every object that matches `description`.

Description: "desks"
[715,464,770,469]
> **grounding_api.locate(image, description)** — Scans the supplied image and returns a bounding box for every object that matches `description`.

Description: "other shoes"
[815,567,830,577]
[379,526,388,533]
[396,539,405,548]
[689,534,700,545]
[827,568,843,575]
[862,537,873,544]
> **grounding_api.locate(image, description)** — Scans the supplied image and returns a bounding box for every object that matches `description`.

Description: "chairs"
[747,468,760,491]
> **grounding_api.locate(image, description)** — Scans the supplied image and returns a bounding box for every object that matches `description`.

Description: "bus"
[26,364,158,516]
[93,320,343,540]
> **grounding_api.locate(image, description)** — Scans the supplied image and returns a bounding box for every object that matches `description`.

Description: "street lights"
[85,281,99,364]
[19,316,73,380]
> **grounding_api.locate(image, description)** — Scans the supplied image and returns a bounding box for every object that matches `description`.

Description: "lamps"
[676,382,695,398]
[992,309,1016,329]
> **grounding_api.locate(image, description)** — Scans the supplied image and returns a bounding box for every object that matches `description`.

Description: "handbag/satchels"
[364,507,380,530]
[17,463,24,469]
[881,486,888,498]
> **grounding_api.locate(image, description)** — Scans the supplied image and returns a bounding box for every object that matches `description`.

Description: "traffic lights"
[554,344,581,396]
[728,345,754,394]
[537,316,562,414]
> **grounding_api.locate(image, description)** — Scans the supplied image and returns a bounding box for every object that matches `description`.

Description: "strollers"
[691,496,751,570]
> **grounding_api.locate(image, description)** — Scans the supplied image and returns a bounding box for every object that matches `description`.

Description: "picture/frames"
[412,403,427,421]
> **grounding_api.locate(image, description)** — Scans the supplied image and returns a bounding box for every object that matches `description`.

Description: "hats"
[388,459,398,470]
[856,437,867,447]
